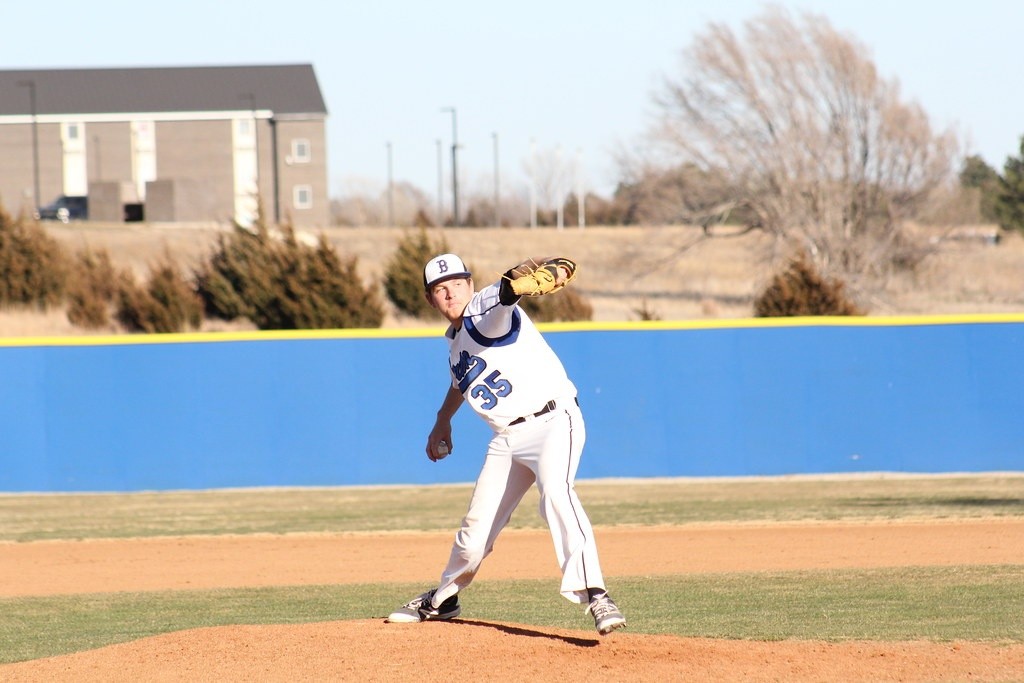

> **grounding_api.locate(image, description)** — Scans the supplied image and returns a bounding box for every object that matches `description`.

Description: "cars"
[35,196,87,222]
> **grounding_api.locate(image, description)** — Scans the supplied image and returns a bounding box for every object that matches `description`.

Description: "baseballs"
[437,441,448,459]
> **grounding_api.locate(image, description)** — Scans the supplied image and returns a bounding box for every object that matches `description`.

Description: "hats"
[422,253,470,292]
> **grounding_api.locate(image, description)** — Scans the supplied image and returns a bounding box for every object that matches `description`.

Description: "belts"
[508,396,579,426]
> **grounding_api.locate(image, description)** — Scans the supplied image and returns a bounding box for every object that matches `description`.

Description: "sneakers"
[387,589,461,622]
[584,590,628,635]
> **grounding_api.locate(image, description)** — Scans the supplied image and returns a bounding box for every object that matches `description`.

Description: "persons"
[385,254,626,635]
